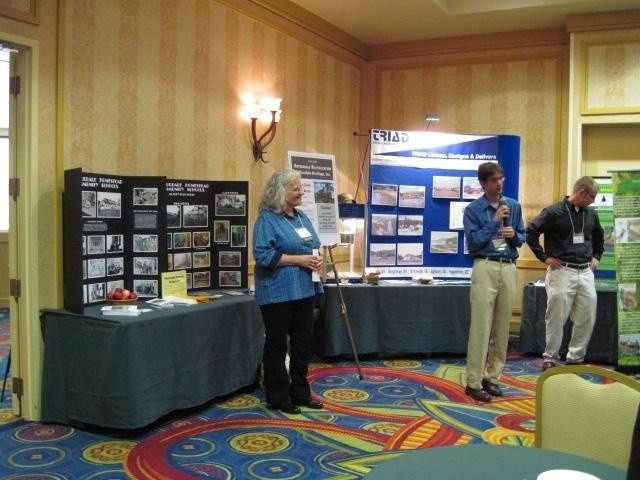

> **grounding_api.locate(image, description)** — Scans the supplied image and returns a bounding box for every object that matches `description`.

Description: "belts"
[482,257,516,263]
[562,263,590,269]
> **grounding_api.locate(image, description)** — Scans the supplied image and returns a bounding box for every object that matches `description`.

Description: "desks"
[361,444,628,480]
[38,291,266,428]
[311,280,473,357]
[518,283,618,362]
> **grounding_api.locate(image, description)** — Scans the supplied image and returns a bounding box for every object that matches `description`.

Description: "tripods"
[323,246,365,383]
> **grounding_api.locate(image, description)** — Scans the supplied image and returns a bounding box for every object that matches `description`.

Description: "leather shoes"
[483,378,502,396]
[465,386,491,401]
[301,397,323,409]
[279,399,301,414]
[543,362,555,370]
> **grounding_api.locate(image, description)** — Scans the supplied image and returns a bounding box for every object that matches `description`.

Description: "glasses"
[486,176,506,183]
[286,184,304,193]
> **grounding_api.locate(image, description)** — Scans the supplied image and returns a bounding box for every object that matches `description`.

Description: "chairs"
[535,365,640,469]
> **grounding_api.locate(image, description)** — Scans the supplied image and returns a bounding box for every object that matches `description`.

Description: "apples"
[108,288,137,300]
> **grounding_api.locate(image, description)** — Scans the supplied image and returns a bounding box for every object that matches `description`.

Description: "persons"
[249,164,329,414]
[463,161,527,403]
[522,174,607,371]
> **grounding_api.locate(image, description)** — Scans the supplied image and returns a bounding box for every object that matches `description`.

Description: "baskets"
[107,296,138,306]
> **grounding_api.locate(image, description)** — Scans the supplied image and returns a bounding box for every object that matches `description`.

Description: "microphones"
[499,198,510,243]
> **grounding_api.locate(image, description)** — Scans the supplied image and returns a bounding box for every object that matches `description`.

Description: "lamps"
[237,93,282,164]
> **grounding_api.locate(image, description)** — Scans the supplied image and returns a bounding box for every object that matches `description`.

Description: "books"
[101,296,199,317]
